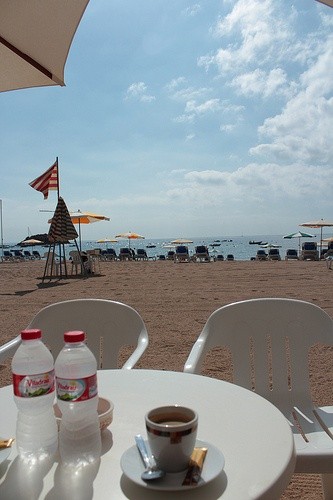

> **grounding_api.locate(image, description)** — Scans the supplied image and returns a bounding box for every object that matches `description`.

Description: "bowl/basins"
[54,397,114,433]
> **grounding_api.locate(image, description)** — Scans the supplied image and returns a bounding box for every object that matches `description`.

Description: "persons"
[324,250,333,272]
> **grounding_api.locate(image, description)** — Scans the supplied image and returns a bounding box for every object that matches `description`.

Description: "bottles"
[11,329,56,459]
[54,331,102,466]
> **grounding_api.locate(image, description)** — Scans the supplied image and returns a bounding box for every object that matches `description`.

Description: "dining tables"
[0,369,299,500]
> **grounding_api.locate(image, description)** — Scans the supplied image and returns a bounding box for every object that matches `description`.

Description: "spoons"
[134,433,165,481]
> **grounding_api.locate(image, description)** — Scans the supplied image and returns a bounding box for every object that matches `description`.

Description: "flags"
[28,161,58,200]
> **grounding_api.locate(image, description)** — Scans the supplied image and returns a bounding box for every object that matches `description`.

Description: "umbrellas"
[49,209,110,274]
[164,239,193,251]
[115,232,145,247]
[284,231,316,254]
[96,237,117,250]
[1,0,90,95]
[21,239,43,255]
[47,197,78,279]
[299,218,333,256]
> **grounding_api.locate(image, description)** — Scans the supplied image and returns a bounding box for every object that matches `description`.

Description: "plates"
[121,438,225,491]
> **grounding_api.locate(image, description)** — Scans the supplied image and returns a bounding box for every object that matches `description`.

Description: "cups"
[145,405,198,473]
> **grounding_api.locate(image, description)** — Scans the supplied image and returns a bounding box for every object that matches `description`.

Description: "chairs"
[183,297,333,499]
[0,297,150,370]
[1,238,333,272]
